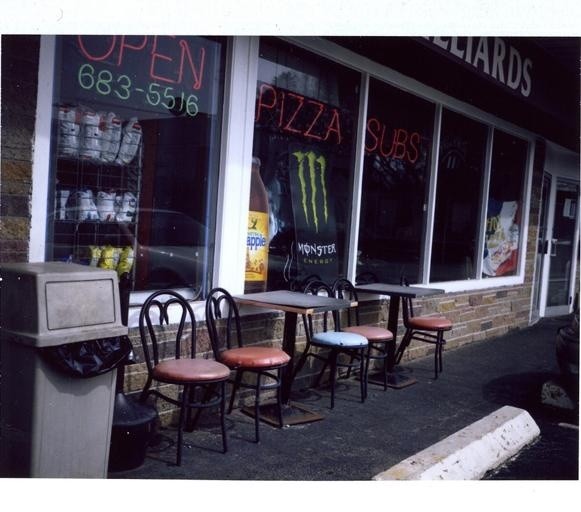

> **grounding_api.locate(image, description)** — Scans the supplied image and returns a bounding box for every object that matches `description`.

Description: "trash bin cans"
[0,261,130,478]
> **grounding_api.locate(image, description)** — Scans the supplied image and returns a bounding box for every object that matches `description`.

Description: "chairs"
[396,273,452,380]
[204,288,292,443]
[138,289,231,466]
[335,277,394,399]
[291,279,369,409]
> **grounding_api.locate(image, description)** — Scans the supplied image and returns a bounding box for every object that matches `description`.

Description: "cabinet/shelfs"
[51,119,144,291]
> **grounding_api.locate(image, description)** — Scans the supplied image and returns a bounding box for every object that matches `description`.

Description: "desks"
[354,282,445,389]
[232,290,358,429]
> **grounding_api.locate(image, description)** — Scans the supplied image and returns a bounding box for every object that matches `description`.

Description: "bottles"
[243,157,270,295]
